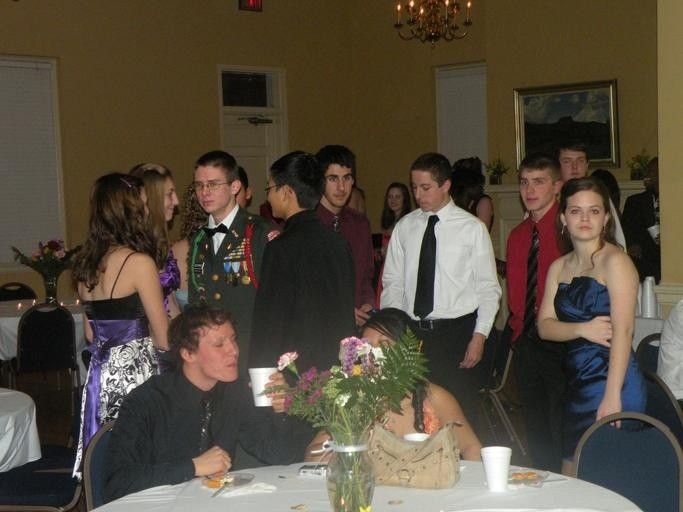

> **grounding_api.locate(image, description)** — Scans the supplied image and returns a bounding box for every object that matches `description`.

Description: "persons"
[314,145,376,327]
[450,157,494,233]
[622,156,661,285]
[94,304,331,504]
[303,307,481,463]
[537,177,647,478]
[523,141,628,255]
[376,183,411,307]
[656,300,683,408]
[130,151,282,378]
[379,152,503,428]
[70,173,169,483]
[245,149,359,374]
[507,153,563,475]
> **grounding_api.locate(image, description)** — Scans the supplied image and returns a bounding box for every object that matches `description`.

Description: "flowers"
[483,152,511,176]
[623,147,651,170]
[10,239,84,301]
[255,322,432,512]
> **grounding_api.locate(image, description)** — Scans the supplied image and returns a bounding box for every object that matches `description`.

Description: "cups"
[646,224,663,245]
[480,445,512,492]
[403,431,431,441]
[245,367,283,409]
[636,276,660,319]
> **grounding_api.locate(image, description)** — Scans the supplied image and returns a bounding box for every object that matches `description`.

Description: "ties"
[414,215,439,319]
[523,227,541,347]
[332,217,341,234]
[200,399,214,437]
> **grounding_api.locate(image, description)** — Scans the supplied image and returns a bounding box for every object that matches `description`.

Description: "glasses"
[263,182,281,193]
[187,182,231,191]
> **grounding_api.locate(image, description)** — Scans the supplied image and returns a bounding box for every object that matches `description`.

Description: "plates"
[510,467,548,482]
[200,471,253,489]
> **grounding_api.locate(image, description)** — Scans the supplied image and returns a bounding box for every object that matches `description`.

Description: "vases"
[325,430,376,512]
[630,170,644,180]
[489,174,503,185]
[42,276,58,304]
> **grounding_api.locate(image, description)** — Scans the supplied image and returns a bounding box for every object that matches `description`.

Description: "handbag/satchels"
[367,421,460,490]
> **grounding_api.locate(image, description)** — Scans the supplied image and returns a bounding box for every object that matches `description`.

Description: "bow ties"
[201,224,228,238]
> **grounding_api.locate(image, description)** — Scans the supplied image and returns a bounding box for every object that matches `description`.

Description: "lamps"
[393,1,473,49]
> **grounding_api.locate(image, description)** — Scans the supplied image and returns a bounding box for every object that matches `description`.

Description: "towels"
[198,477,277,498]
[484,468,569,490]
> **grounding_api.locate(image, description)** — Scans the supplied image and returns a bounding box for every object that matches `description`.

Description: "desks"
[484,180,646,263]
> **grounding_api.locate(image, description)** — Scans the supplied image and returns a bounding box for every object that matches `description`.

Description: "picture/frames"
[513,78,620,172]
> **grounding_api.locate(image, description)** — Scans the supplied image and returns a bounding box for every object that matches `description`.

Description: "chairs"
[5,302,79,416]
[571,411,683,512]
[478,336,526,457]
[635,334,683,452]
[0,417,117,512]
[0,283,39,301]
